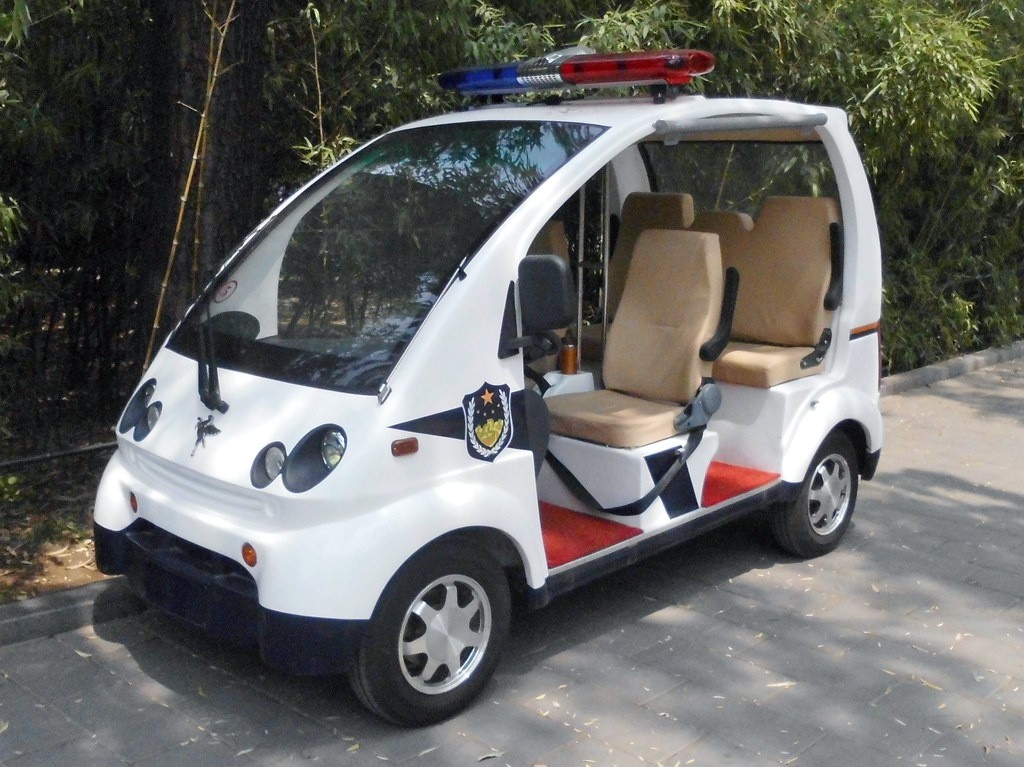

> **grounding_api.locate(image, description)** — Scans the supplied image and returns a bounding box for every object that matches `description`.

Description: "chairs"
[527,191,839,449]
